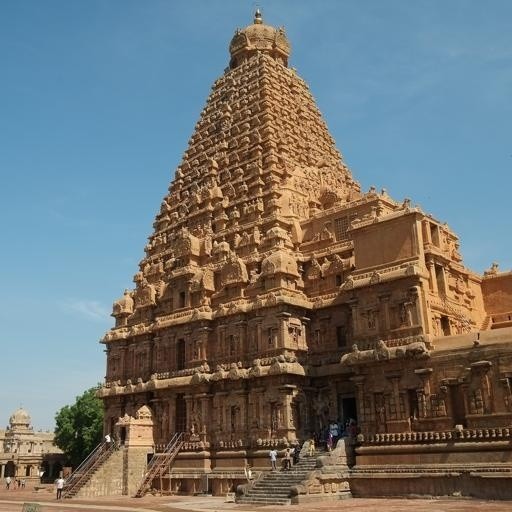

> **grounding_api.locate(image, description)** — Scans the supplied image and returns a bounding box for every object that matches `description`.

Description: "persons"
[105,432,111,450]
[6,477,12,490]
[293,444,300,464]
[269,448,278,471]
[56,477,64,500]
[325,416,356,451]
[284,448,290,470]
[14,480,17,490]
[22,479,26,488]
[18,480,20,487]
